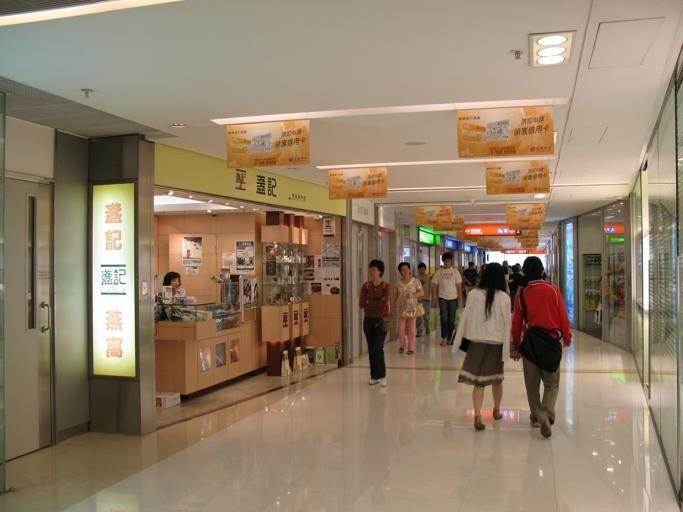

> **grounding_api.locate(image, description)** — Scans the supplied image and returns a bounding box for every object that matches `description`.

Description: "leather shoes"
[399,346,404,353]
[408,351,413,354]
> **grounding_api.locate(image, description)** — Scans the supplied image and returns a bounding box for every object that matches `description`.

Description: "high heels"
[474,422,486,432]
[493,411,503,420]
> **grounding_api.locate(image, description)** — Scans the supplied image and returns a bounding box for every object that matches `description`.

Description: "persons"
[411,263,433,338]
[459,252,547,305]
[158,271,181,295]
[450,261,521,430]
[508,254,572,438]
[390,262,424,354]
[429,252,462,347]
[358,259,393,387]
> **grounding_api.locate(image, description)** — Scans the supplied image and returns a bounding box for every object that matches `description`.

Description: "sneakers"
[380,378,386,387]
[531,422,540,427]
[536,411,551,439]
[440,341,447,346]
[368,379,379,385]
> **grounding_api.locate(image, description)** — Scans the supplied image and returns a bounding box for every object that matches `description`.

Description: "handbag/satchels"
[399,303,426,318]
[518,326,563,372]
